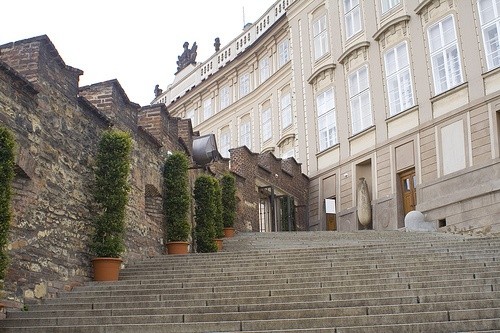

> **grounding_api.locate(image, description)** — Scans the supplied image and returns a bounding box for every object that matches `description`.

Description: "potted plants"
[214,178,224,250]
[163,151,190,255]
[91,130,132,282]
[220,175,236,237]
